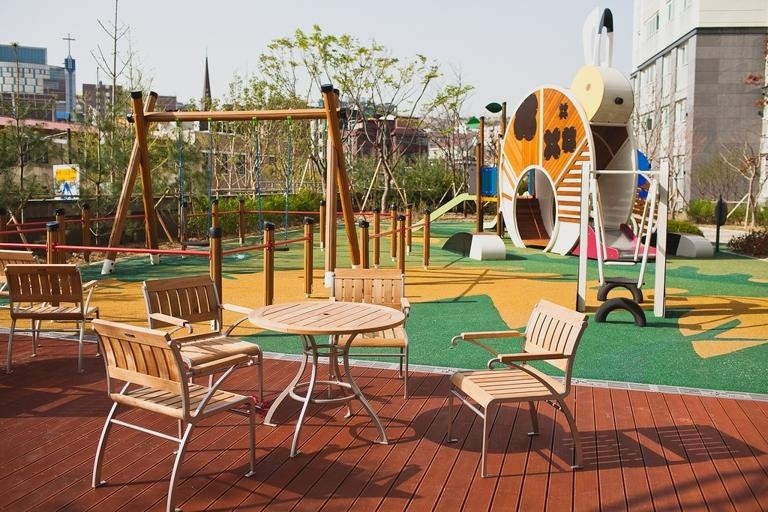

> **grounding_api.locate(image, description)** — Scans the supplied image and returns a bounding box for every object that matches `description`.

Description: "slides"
[412,192,469,232]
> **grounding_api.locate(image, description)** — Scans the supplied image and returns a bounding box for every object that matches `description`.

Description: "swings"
[591,173,658,284]
[252,116,292,251]
[176,118,212,246]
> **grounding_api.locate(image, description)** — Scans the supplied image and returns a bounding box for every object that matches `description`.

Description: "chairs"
[327,267,411,400]
[4,263,100,375]
[445,299,589,478]
[0,250,56,348]
[142,275,264,410]
[90,317,258,512]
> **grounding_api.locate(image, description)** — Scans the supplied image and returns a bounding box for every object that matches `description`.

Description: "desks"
[247,301,406,461]
